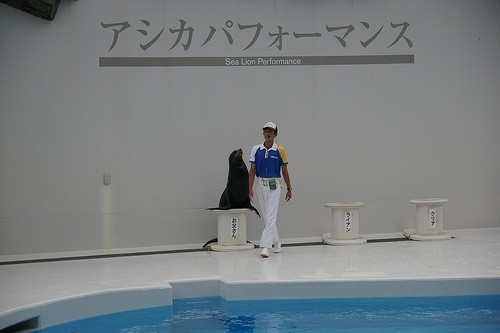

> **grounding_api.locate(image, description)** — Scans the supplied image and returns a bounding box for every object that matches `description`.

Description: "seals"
[205,148,261,219]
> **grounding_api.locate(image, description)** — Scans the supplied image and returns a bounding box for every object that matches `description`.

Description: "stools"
[322,202,367,246]
[403,198,452,241]
[207,209,255,252]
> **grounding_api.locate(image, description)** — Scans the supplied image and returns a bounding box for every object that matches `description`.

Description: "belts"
[257,180,281,185]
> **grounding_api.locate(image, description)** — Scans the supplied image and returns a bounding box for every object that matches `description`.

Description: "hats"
[262,122,278,133]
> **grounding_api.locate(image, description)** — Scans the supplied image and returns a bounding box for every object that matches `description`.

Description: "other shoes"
[261,250,269,257]
[274,239,282,253]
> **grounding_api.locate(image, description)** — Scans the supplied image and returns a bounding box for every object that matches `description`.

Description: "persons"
[248,122,293,257]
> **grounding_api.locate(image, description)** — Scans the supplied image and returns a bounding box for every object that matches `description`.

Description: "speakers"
[0,0,61,21]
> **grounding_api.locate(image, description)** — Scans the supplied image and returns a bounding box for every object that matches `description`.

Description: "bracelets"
[286,188,292,190]
